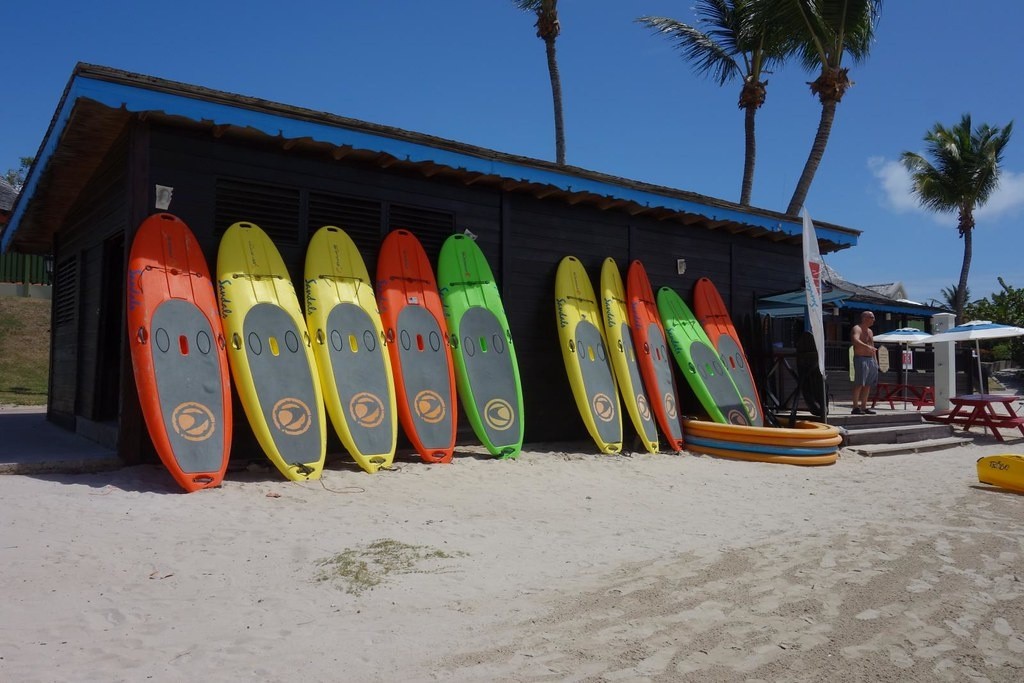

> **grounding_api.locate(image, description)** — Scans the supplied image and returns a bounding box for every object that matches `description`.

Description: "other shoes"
[851,407,865,414]
[863,409,876,414]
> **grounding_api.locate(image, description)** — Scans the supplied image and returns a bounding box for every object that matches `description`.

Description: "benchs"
[948,412,1024,436]
[864,396,936,410]
[924,414,1022,443]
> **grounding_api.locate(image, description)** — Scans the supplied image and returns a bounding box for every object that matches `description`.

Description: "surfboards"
[625,260,687,454]
[554,255,624,456]
[374,228,460,465]
[216,220,329,483]
[437,232,526,461]
[693,277,765,428]
[600,256,661,456]
[123,212,234,492]
[303,225,400,475]
[655,286,755,427]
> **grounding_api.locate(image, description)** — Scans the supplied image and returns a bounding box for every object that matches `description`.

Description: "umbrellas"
[908,318,1024,438]
[873,327,933,410]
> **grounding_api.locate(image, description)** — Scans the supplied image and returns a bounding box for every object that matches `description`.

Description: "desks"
[875,383,934,410]
[948,394,1020,441]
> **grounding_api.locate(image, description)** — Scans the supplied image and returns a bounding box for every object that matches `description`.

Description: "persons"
[850,311,879,414]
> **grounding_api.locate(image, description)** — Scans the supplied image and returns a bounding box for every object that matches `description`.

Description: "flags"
[801,203,826,378]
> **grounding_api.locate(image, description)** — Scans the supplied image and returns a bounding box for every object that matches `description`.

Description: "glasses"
[864,317,875,319]
